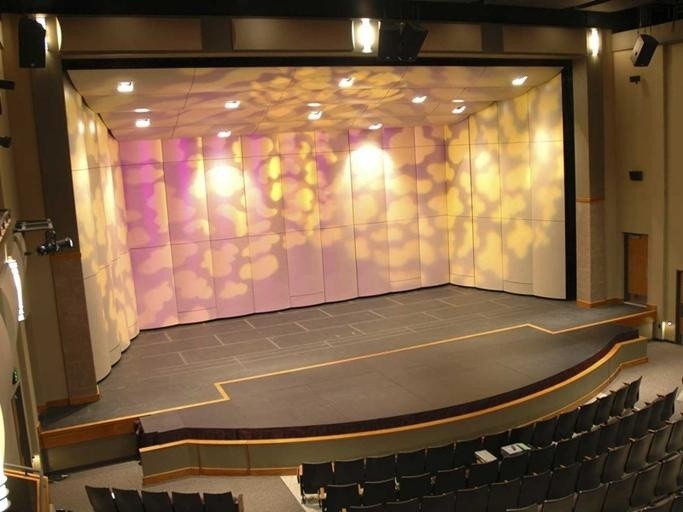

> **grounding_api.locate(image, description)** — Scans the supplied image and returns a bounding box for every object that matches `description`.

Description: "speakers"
[18,19,45,69]
[629,34,659,67]
[378,19,428,61]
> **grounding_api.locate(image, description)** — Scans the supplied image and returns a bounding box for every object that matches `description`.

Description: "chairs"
[84,484,245,512]
[296,376,683,512]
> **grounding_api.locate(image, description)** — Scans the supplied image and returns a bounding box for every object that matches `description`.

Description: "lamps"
[586,12,608,54]
[25,230,75,259]
[350,17,380,54]
